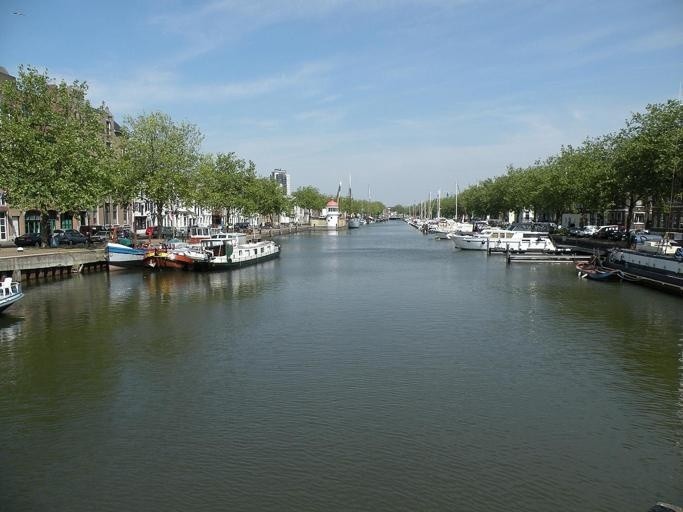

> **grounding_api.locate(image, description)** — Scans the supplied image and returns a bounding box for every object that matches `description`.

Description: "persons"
[591,255,600,267]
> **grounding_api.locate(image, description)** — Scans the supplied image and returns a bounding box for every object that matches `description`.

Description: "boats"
[447,224,572,252]
[348,213,390,229]
[576,256,622,284]
[105,226,280,270]
[0,276,24,312]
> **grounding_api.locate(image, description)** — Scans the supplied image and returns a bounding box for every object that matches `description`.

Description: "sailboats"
[602,81,683,290]
[402,179,473,239]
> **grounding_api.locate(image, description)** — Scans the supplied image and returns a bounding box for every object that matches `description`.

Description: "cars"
[14,233,52,246]
[489,218,562,231]
[146,221,298,238]
[51,224,133,247]
[568,224,683,255]
